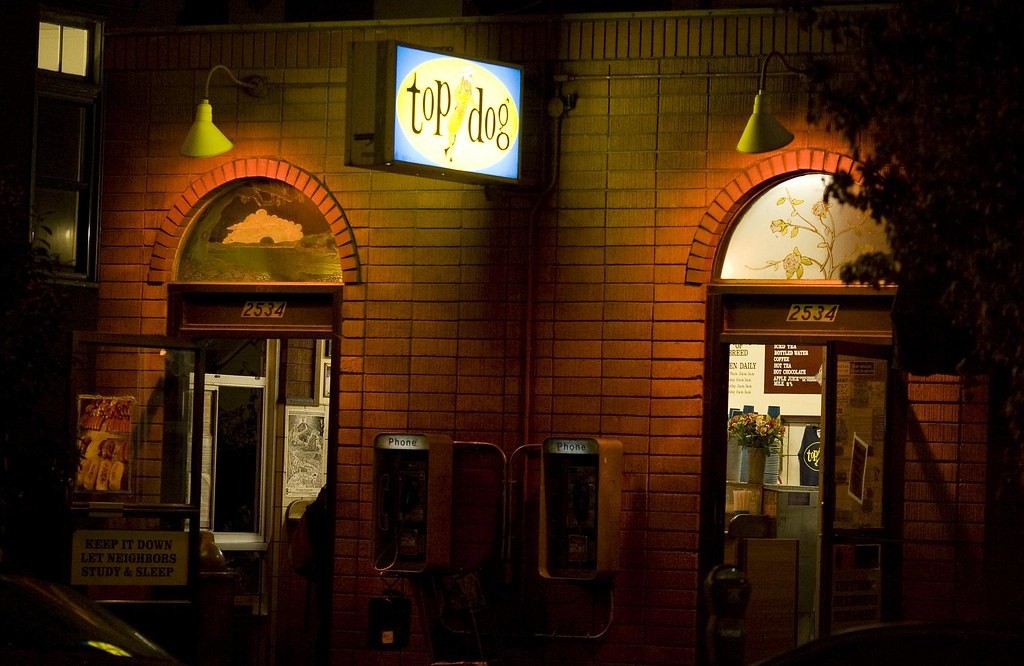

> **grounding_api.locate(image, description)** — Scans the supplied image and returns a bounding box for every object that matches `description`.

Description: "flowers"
[726,413,786,457]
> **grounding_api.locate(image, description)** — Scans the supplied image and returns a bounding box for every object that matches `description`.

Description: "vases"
[745,447,766,485]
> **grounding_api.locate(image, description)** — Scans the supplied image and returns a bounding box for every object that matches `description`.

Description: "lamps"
[179,65,270,158]
[737,52,810,153]
